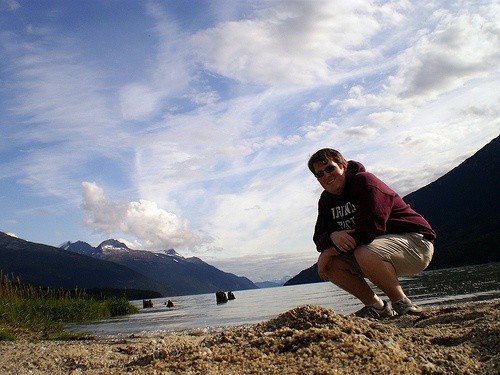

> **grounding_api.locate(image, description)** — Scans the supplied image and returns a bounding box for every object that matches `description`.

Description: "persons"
[308,149,436,315]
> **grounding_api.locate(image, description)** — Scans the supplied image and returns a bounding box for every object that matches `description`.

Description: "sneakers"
[349,300,392,318]
[391,295,420,314]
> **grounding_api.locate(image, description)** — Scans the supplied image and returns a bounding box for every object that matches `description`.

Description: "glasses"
[314,165,337,177]
